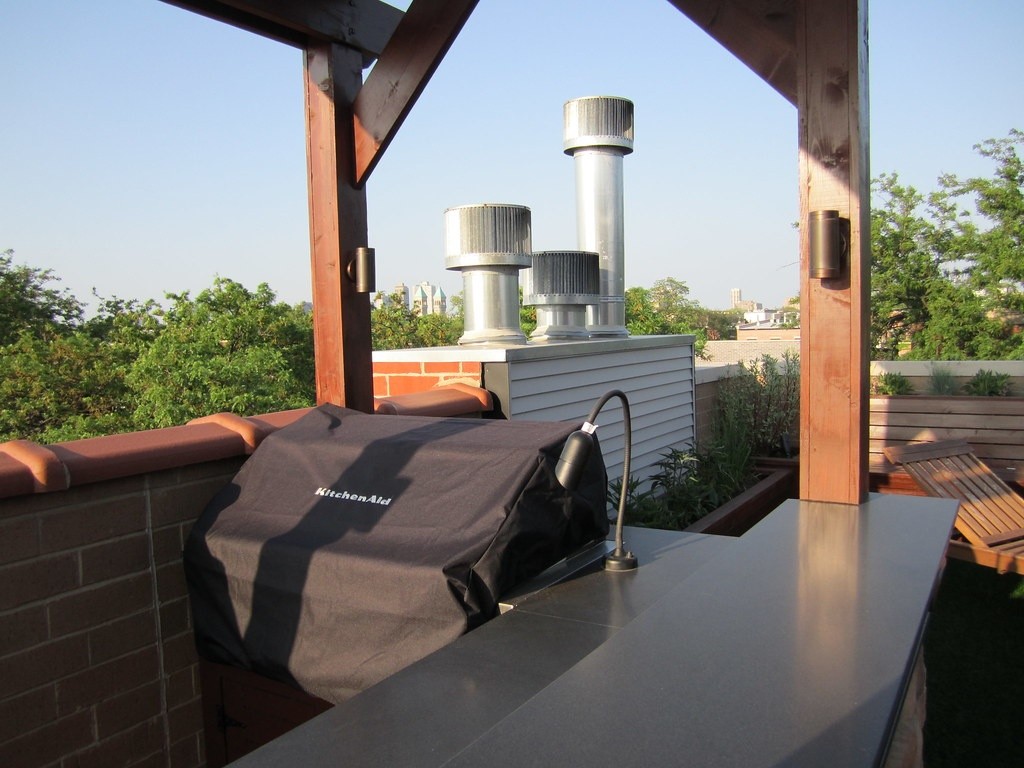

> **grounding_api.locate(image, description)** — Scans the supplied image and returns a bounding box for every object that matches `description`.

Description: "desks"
[215,489,962,768]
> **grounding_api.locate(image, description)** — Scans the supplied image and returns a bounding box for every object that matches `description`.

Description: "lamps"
[349,247,377,293]
[555,388,639,571]
[808,208,850,279]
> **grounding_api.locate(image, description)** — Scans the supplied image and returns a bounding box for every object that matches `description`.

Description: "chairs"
[886,436,1024,575]
